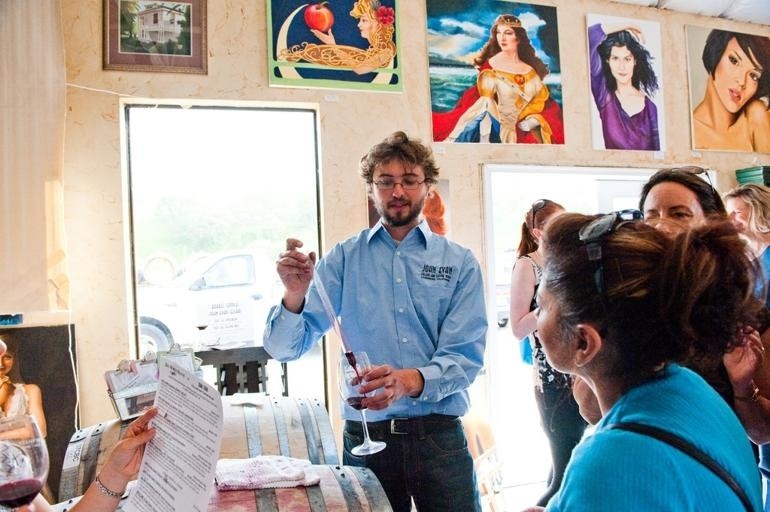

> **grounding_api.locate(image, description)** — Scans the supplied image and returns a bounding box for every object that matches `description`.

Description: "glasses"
[579,208,643,297]
[533,199,547,228]
[650,165,706,179]
[374,181,422,189]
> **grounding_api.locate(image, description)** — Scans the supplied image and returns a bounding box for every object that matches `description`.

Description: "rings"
[761,345,765,352]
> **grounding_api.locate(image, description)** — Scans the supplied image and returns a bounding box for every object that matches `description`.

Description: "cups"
[0,414,50,512]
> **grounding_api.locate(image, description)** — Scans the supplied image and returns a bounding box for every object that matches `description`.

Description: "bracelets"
[732,382,761,402]
[95,475,126,497]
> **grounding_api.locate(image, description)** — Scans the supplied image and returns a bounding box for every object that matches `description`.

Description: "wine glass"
[337,352,387,455]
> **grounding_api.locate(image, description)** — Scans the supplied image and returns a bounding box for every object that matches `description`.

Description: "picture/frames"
[100,0,211,75]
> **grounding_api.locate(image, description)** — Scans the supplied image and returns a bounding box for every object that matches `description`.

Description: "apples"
[304,3,335,32]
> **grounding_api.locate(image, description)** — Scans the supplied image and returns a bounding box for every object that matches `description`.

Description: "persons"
[520,208,766,511]
[588,21,661,152]
[263,131,490,511]
[511,198,587,505]
[0,334,59,504]
[573,165,770,449]
[431,12,564,144]
[721,182,770,511]
[692,29,769,154]
[0,406,158,511]
[307,1,395,76]
